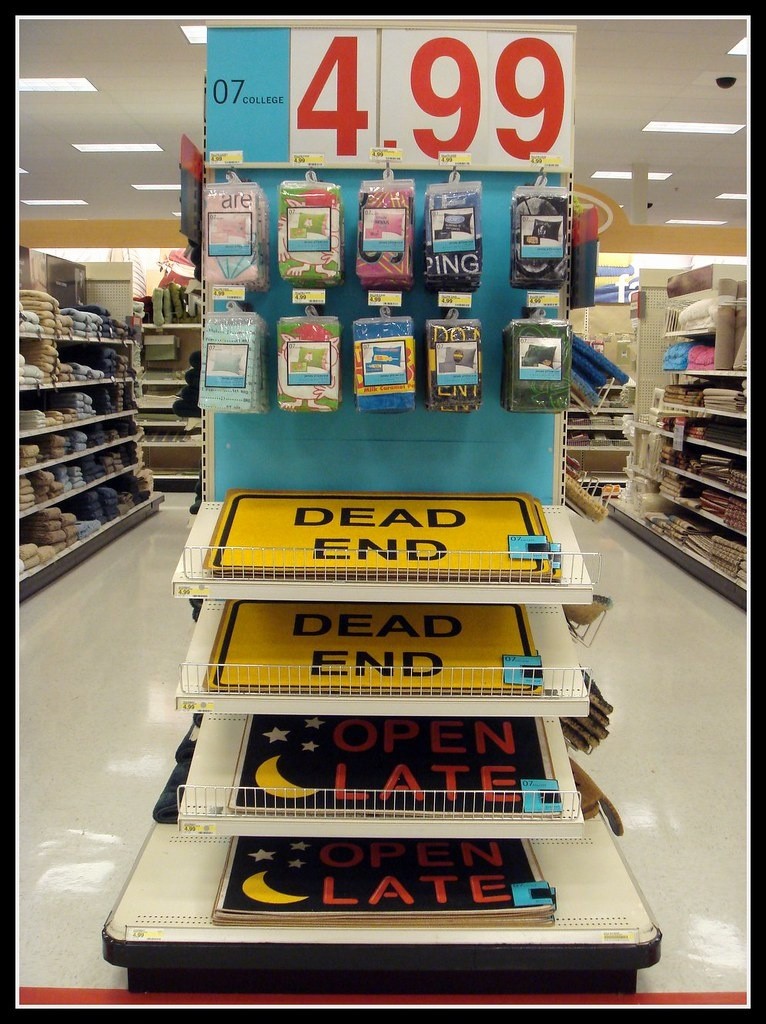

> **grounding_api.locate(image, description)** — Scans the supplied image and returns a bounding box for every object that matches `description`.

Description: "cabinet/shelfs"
[567,384,634,496]
[602,328,747,611]
[101,485,663,994]
[19,333,165,603]
[134,323,202,492]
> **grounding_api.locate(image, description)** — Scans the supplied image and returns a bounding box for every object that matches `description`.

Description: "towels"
[642,381,747,583]
[19,289,154,574]
